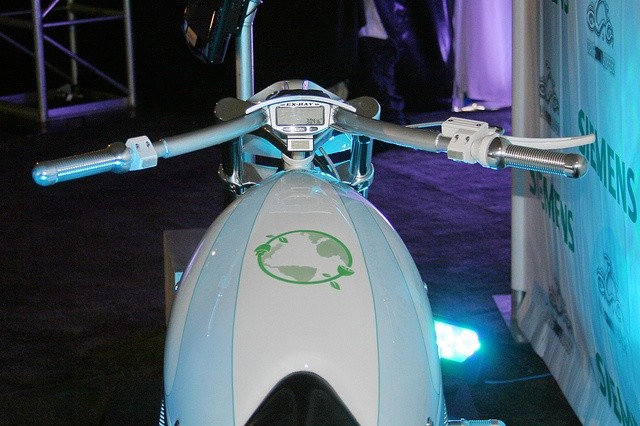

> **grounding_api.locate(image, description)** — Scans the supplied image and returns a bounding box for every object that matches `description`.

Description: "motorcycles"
[32,79,596,426]
